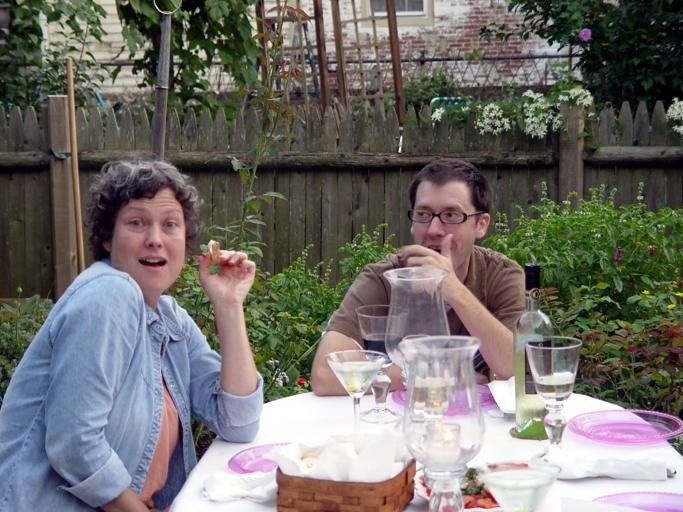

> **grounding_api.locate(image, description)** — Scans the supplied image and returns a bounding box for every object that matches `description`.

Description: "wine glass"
[397,335,486,510]
[383,266,451,463]
[354,304,404,425]
[325,349,388,455]
[475,463,562,511]
[524,335,583,467]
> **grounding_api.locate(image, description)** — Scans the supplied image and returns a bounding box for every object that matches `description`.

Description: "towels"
[204,471,279,504]
[547,455,668,481]
[487,375,516,418]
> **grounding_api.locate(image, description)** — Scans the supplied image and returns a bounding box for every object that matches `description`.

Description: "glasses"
[407,209,486,225]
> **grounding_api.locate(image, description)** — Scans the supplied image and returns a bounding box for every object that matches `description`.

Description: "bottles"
[515,263,554,432]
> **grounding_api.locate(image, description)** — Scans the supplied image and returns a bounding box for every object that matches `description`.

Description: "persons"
[310,159,527,397]
[0,158,263,511]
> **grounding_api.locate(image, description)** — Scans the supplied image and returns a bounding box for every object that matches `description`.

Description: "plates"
[590,490,683,512]
[391,382,495,416]
[228,441,290,476]
[568,408,683,444]
